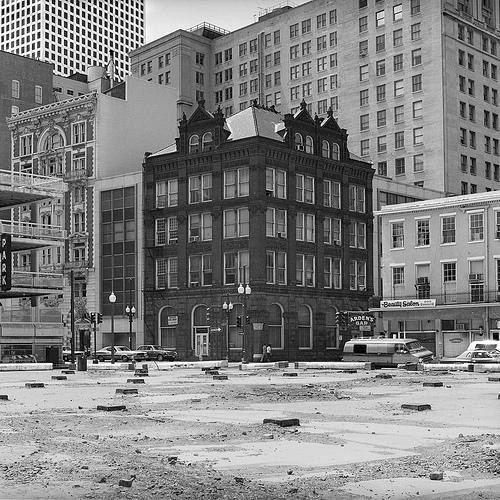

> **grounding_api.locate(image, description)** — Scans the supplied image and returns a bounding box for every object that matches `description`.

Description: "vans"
[467,339,500,352]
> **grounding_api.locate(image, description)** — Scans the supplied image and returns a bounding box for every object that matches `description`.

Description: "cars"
[62,346,84,361]
[92,345,149,363]
[437,348,500,364]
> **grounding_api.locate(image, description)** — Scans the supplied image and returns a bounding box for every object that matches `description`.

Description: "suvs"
[134,345,178,361]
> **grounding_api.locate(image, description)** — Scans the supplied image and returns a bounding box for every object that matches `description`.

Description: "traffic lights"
[237,316,241,327]
[335,312,341,325]
[98,312,103,324]
[91,312,96,324]
[342,312,349,324]
[246,315,250,325]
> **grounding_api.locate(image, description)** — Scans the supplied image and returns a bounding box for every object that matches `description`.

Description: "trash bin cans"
[78,355,87,371]
[46,345,60,366]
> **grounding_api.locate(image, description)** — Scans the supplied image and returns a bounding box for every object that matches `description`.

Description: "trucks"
[340,338,436,365]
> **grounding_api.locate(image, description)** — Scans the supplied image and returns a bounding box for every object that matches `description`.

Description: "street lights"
[223,301,233,362]
[238,283,252,364]
[109,292,117,365]
[125,305,136,349]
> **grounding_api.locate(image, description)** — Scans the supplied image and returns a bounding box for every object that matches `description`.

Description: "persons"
[260,344,268,362]
[264,343,273,362]
